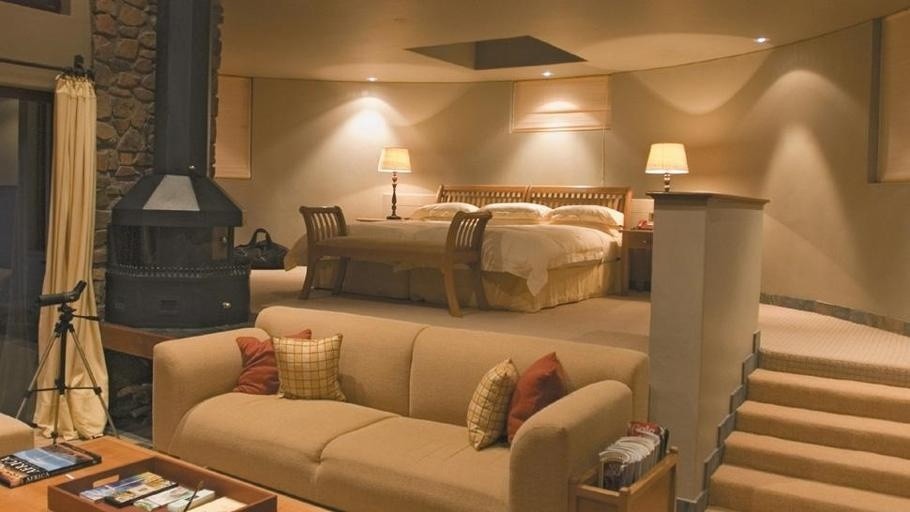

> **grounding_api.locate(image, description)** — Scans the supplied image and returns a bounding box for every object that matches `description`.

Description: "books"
[0,441,102,488]
[597,421,669,490]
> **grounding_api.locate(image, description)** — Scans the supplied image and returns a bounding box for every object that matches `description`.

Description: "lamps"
[378,146,413,220]
[645,143,690,192]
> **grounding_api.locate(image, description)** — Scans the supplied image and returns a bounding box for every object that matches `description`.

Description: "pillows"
[413,202,479,219]
[466,358,519,450]
[272,335,347,402]
[477,202,551,221]
[507,352,567,446]
[546,205,625,225]
[233,329,312,395]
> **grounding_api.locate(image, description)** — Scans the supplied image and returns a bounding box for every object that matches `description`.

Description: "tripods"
[15,304,120,444]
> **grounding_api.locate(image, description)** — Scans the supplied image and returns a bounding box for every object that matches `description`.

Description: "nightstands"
[618,229,651,291]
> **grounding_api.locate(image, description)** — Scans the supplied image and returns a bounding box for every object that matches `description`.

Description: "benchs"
[299,205,492,318]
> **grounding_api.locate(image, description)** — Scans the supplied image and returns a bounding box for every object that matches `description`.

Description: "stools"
[0,413,34,458]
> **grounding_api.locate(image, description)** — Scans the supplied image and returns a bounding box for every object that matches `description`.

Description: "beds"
[312,185,633,313]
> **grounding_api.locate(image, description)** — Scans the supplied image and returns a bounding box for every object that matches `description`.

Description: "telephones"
[635,219,652,230]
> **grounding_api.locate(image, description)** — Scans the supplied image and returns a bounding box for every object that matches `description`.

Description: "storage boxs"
[569,446,681,512]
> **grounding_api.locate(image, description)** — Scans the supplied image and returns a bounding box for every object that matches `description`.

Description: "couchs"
[152,306,651,512]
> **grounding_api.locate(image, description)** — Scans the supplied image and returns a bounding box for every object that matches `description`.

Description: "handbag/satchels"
[236,228,288,269]
